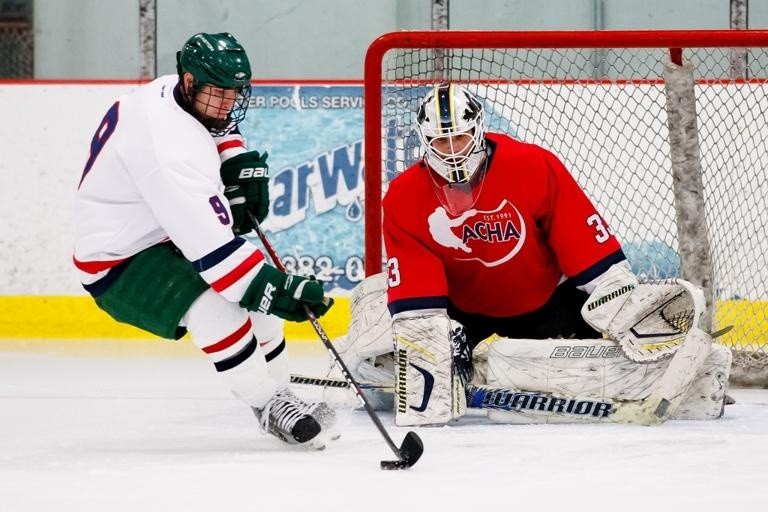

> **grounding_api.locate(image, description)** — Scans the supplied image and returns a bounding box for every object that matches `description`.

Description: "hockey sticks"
[288,326,712,426]
[246,206,424,466]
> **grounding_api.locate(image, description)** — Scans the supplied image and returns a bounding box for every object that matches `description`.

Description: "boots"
[252,387,335,446]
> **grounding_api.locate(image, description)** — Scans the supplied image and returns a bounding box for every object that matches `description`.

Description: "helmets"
[176,32,252,93]
[414,83,487,186]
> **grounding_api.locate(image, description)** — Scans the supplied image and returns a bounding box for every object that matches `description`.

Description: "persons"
[71,32,335,447]
[327,81,734,427]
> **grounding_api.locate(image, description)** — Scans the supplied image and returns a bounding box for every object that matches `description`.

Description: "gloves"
[220,151,270,236]
[239,263,334,322]
[391,308,474,427]
[581,265,705,364]
